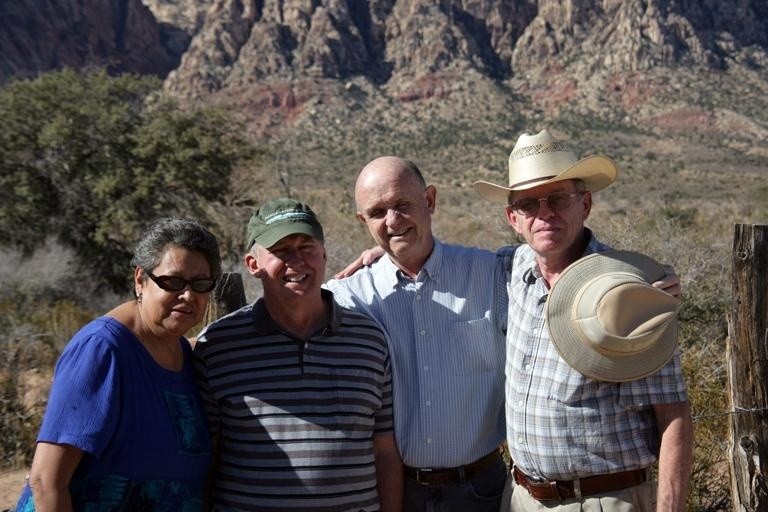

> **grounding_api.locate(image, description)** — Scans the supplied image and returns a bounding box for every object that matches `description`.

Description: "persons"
[13,215,223,512]
[188,196,405,512]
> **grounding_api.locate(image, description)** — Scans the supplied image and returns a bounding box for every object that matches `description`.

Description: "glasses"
[511,191,578,215]
[147,270,216,293]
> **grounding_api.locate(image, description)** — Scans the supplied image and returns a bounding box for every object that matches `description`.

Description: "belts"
[413,449,499,485]
[514,466,646,500]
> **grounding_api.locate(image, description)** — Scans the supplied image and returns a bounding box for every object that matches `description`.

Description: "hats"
[248,198,324,248]
[474,129,617,201]
[547,249,681,383]
